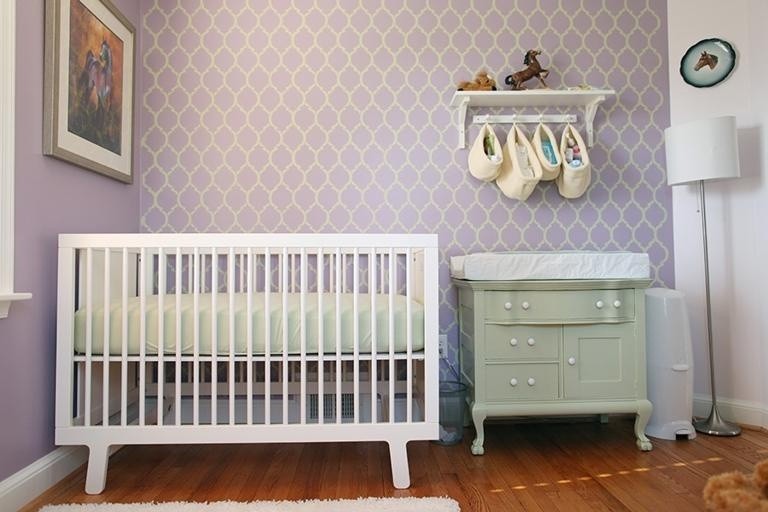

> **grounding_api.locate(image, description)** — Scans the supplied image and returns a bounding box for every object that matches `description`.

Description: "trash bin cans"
[430,381,466,447]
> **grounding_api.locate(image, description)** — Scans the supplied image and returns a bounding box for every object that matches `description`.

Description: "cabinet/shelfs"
[452,280,654,457]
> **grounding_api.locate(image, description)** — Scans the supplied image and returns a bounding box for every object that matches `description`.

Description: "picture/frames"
[42,0,137,186]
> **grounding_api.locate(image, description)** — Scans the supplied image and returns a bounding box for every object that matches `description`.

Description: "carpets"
[38,495,461,512]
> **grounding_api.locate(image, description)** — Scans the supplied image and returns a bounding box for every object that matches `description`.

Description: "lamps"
[664,115,742,437]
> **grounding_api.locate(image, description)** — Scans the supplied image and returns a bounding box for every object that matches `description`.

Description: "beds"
[57,232,441,495]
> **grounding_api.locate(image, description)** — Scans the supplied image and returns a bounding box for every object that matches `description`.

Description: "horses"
[504,49,550,90]
[693,50,718,72]
